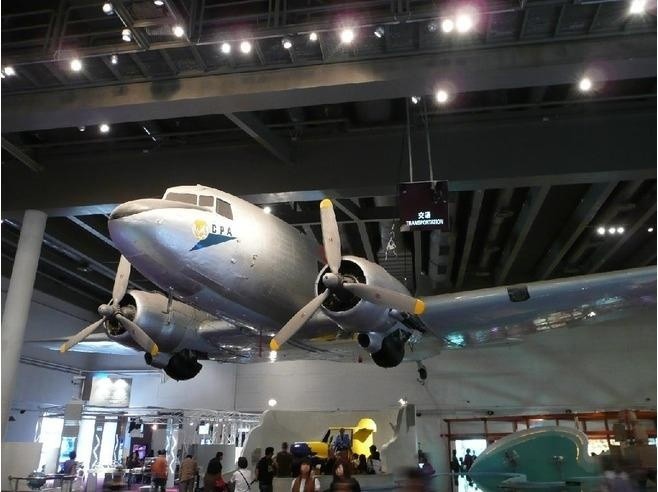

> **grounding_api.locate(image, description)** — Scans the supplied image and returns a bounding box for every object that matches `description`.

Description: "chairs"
[103,469,132,491]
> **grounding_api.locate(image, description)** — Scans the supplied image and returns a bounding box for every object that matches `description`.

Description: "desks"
[10,473,76,492]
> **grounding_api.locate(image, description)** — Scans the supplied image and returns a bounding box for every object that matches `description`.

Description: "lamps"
[372,21,389,41]
[279,37,294,50]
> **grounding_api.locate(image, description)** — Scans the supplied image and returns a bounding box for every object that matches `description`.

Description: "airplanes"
[23,184,657,382]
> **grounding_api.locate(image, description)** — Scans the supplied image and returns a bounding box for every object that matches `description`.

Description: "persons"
[448,447,478,473]
[228,456,252,492]
[254,427,381,492]
[60,449,80,490]
[420,458,434,474]
[202,451,227,492]
[453,473,474,487]
[124,447,201,492]
